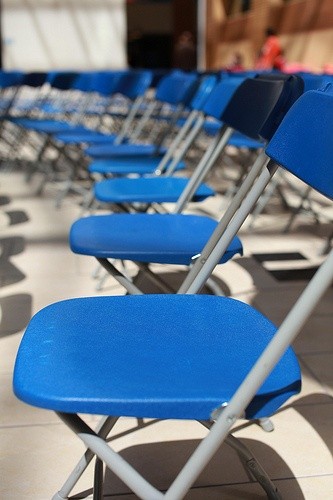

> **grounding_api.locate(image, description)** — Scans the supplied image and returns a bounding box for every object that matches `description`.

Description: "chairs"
[0,69,333,499]
